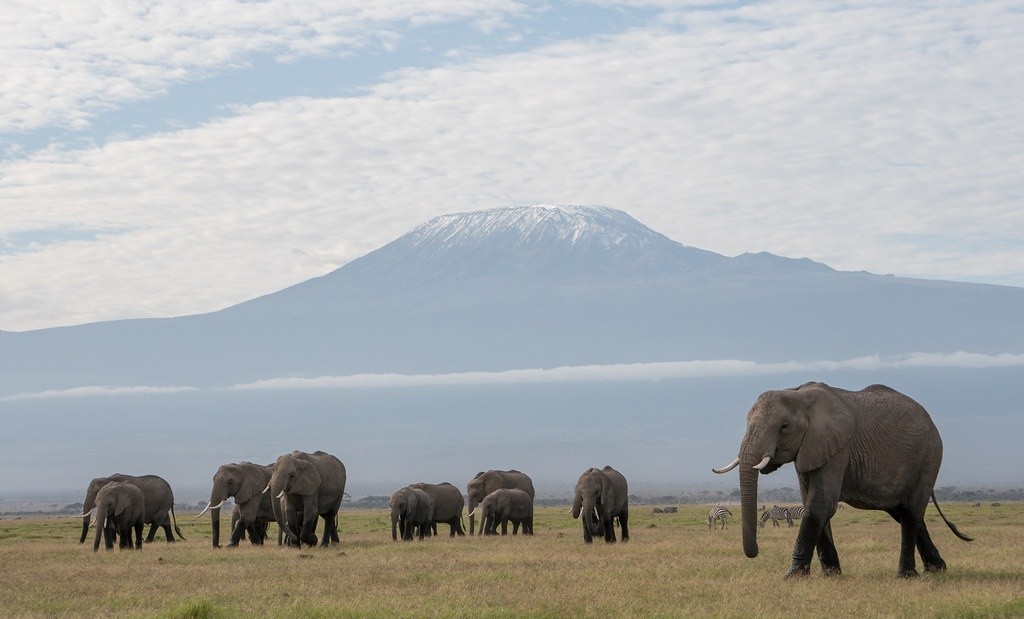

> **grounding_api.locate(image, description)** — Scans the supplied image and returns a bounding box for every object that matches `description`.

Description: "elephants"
[566,466,628,545]
[196,450,347,549]
[72,472,190,551]
[710,381,975,578]
[387,469,535,541]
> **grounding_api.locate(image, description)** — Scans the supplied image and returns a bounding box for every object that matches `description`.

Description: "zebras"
[757,504,806,530]
[707,505,733,532]
